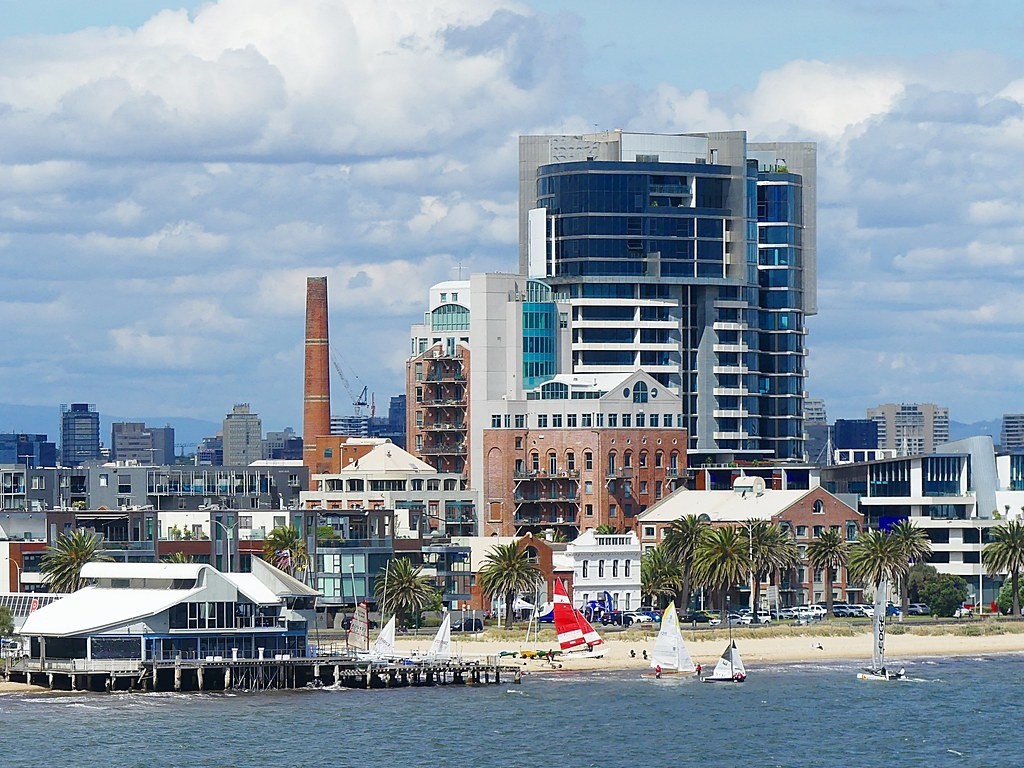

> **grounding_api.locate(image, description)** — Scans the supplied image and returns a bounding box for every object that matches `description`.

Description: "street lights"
[468,519,530,627]
[947,520,1013,615]
[5,557,20,593]
[718,516,784,613]
[205,519,250,573]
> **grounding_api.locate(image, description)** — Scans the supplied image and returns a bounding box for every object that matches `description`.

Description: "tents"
[500,597,534,615]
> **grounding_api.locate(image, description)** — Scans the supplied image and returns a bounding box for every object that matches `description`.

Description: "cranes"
[329,345,369,416]
[174,443,199,456]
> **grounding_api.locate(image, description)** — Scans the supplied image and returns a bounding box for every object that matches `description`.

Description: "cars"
[908,603,931,616]
[834,605,864,618]
[851,605,875,617]
[741,610,771,624]
[642,611,663,622]
[696,611,719,618]
[679,611,709,623]
[708,614,743,626]
[770,605,847,620]
[624,612,653,623]
[954,606,969,617]
[451,618,483,632]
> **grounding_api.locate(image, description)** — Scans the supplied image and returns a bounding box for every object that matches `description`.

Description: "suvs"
[601,612,634,627]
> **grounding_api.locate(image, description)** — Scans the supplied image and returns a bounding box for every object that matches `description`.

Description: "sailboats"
[554,577,611,658]
[348,600,452,663]
[704,639,747,682]
[641,599,698,678]
[520,578,563,657]
[861,577,906,680]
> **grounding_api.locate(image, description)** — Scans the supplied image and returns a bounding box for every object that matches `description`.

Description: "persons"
[546,649,552,663]
[656,664,661,679]
[485,609,496,620]
[896,667,905,677]
[696,663,701,675]
[811,643,823,651]
